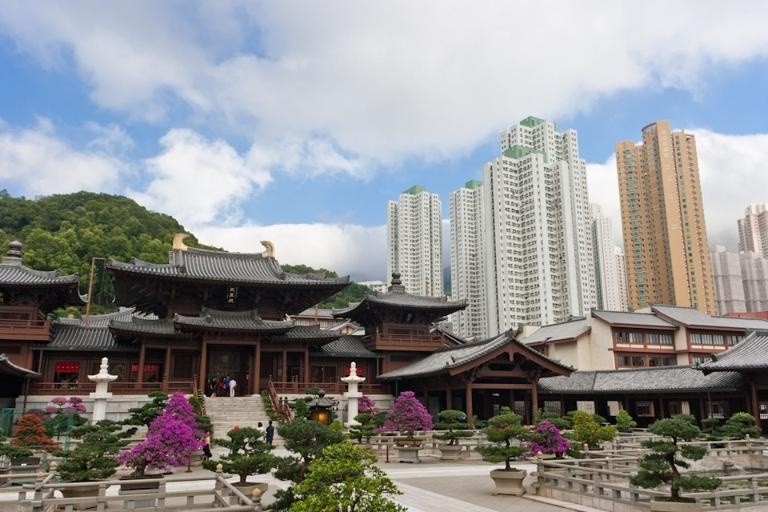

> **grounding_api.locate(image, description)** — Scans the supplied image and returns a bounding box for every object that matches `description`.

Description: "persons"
[55,374,79,390]
[266,420,274,453]
[232,423,240,430]
[256,422,266,442]
[205,371,237,397]
[202,430,212,461]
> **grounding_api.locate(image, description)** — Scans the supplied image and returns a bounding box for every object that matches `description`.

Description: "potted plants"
[385,390,616,499]
[0,397,281,511]
[629,411,762,512]
